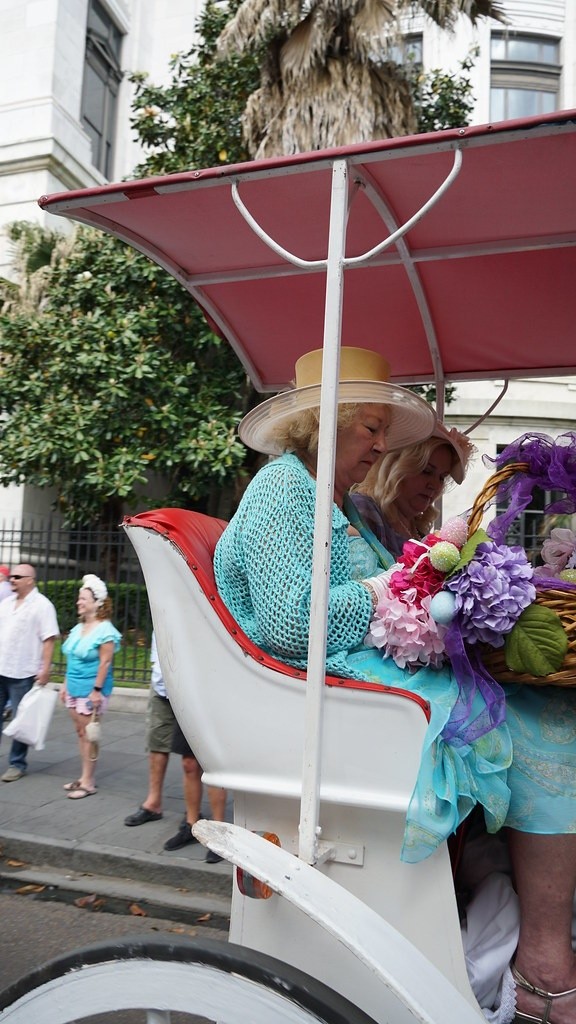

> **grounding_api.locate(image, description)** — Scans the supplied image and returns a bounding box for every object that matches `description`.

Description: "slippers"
[64,780,97,790]
[66,788,96,799]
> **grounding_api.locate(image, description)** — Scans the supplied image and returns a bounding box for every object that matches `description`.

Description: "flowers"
[365,532,533,674]
[537,524,576,574]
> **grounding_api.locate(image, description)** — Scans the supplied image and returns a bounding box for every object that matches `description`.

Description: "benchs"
[117,511,470,1000]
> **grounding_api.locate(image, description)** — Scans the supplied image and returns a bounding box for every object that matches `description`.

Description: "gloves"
[363,562,406,605]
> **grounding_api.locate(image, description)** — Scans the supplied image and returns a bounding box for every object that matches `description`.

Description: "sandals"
[510,964,576,1024]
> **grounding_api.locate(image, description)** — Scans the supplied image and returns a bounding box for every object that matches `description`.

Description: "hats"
[432,422,479,485]
[237,346,437,456]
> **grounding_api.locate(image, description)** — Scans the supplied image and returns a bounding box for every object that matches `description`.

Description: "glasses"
[8,575,29,579]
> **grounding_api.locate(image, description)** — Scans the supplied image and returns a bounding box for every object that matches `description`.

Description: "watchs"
[94,686,103,691]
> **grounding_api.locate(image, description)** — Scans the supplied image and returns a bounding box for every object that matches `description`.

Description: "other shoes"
[164,823,199,851]
[2,767,25,781]
[206,851,224,863]
[125,807,162,826]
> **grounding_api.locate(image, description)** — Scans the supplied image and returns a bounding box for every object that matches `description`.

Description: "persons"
[351,421,477,563]
[60,574,122,798]
[0,564,60,781]
[213,347,576,1024]
[124,630,227,862]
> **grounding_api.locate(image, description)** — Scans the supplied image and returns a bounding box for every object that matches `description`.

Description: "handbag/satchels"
[2,686,58,750]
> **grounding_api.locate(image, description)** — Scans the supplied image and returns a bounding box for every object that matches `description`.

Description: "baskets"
[462,462,576,687]
